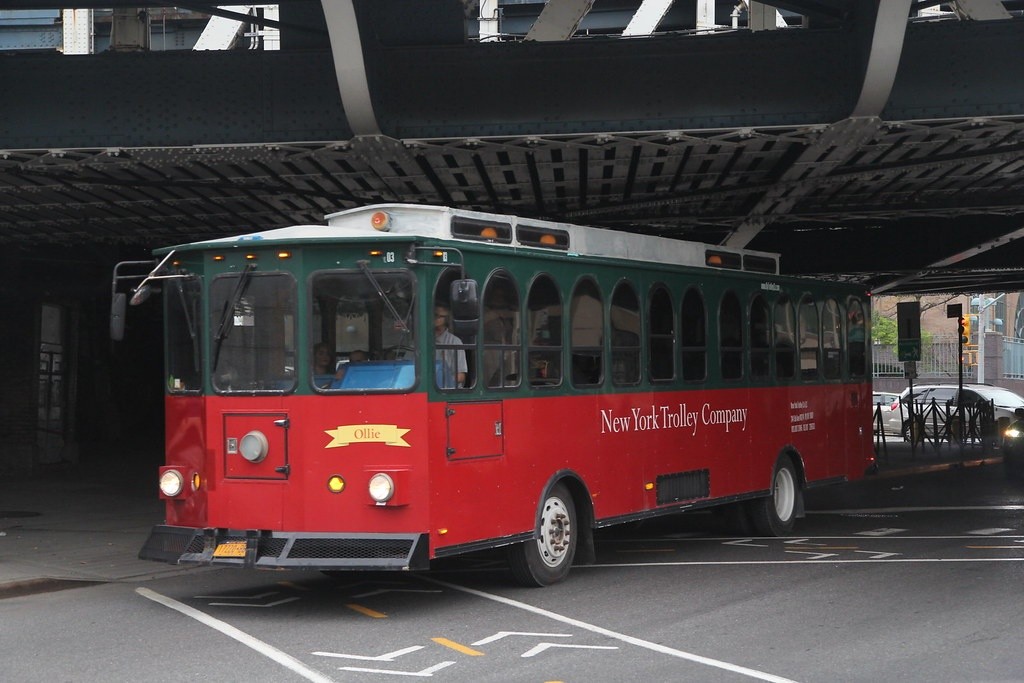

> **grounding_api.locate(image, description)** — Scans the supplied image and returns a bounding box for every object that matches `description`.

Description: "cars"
[872,390,903,436]
[1003,406,1024,480]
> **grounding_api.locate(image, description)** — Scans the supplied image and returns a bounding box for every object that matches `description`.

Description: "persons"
[404,299,468,389]
[335,350,367,380]
[313,341,334,374]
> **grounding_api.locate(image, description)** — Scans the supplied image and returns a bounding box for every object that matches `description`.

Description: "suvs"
[888,380,1024,443]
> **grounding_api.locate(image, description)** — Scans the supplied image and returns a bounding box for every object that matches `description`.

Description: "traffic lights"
[958,317,971,346]
[958,345,978,369]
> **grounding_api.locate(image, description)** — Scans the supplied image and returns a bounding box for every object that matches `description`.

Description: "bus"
[108,200,875,593]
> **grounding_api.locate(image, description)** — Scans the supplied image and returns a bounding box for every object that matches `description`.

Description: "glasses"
[434,313,448,320]
